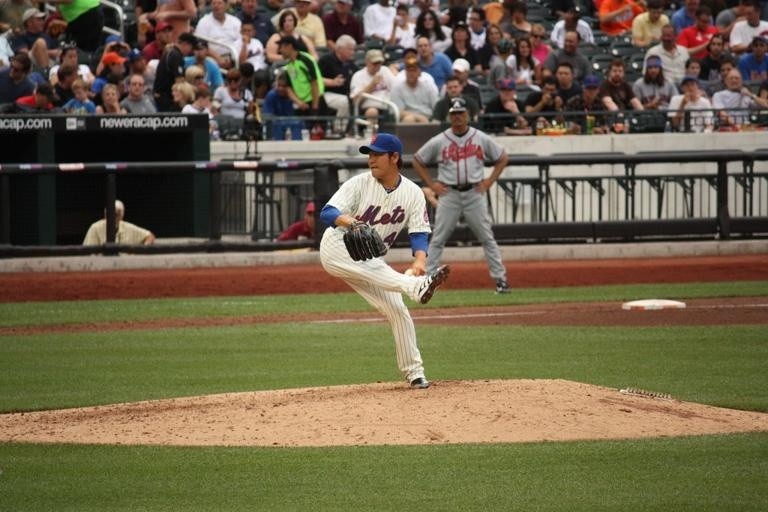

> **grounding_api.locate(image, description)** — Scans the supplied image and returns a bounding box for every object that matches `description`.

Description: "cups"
[583,114,596,134]
[536,118,544,135]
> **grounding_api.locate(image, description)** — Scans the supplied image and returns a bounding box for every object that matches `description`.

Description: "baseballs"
[405,268,412,275]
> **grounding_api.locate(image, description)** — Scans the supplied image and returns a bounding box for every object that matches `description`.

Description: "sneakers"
[494,279,511,296]
[415,263,451,305]
[409,375,430,389]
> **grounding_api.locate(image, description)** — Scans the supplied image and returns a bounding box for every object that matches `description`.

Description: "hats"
[301,199,315,214]
[447,96,467,114]
[679,76,697,85]
[274,34,296,45]
[582,73,599,89]
[357,132,403,155]
[365,47,384,65]
[101,50,128,66]
[176,30,203,53]
[21,6,48,23]
[153,19,174,34]
[497,77,515,91]
[127,47,146,62]
[451,56,471,73]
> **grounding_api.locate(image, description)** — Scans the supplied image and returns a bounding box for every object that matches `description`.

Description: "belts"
[447,183,473,194]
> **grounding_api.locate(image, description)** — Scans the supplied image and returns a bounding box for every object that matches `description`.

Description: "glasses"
[192,75,205,80]
[530,33,545,40]
[226,76,240,84]
[422,16,434,21]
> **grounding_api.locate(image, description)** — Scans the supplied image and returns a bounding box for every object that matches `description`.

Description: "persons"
[274,202,315,241]
[80,199,155,258]
[409,97,510,297]
[318,133,450,387]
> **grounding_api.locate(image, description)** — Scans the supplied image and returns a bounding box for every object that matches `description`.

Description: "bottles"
[664,121,671,132]
[285,129,291,140]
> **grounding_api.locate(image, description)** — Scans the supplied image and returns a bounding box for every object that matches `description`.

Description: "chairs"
[574,31,655,84]
[469,71,534,107]
[525,2,594,34]
[352,36,407,69]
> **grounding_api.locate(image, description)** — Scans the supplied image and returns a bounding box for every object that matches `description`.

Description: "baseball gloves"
[343,220,386,261]
